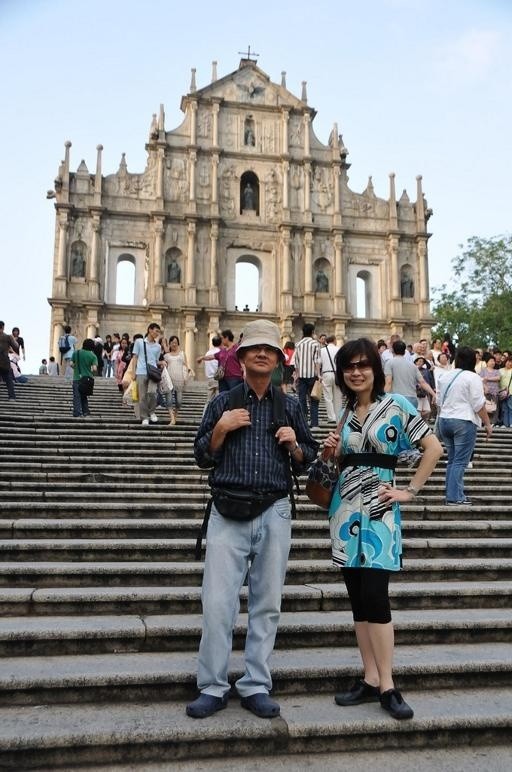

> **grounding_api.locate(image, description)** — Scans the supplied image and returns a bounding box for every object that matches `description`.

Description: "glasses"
[341,360,373,373]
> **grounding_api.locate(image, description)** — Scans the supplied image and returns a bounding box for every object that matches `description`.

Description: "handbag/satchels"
[309,378,324,402]
[146,363,163,383]
[483,400,498,414]
[181,365,190,383]
[303,445,341,509]
[495,387,510,402]
[79,375,95,396]
[213,366,225,381]
[121,381,139,404]
[210,484,282,522]
[155,365,174,394]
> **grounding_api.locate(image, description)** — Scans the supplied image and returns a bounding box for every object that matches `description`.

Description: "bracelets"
[290,441,299,455]
[406,484,419,497]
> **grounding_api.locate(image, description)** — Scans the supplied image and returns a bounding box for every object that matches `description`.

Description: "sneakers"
[380,687,414,720]
[398,453,423,468]
[150,412,159,422]
[446,497,473,506]
[334,678,381,707]
[140,417,150,426]
[487,421,512,429]
[240,691,280,719]
[186,693,229,719]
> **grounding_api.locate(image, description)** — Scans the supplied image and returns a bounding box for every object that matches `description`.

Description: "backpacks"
[58,335,71,353]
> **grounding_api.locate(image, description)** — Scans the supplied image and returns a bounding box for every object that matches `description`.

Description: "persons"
[183,319,319,718]
[235,305,259,312]
[313,336,444,720]
[243,183,254,210]
[433,344,493,506]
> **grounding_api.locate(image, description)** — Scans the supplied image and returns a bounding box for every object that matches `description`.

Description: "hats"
[236,319,285,363]
